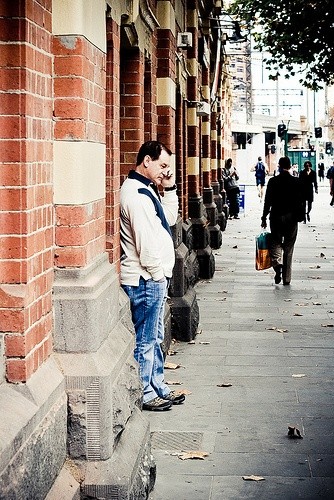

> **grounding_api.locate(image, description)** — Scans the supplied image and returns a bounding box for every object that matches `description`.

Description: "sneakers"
[163,392,185,404]
[143,397,172,411]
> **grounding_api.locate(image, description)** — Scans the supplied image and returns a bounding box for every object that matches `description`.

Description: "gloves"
[261,221,267,229]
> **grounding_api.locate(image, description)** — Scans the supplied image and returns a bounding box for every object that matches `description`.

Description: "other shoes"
[303,218,306,224]
[274,275,281,284]
[234,216,240,219]
[307,213,310,221]
[229,218,232,220]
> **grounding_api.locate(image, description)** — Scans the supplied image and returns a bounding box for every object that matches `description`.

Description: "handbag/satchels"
[255,227,273,271]
[226,186,240,197]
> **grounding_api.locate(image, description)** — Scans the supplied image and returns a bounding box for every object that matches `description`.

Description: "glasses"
[306,165,311,167]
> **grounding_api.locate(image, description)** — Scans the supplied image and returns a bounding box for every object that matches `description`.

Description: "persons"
[326,162,334,206]
[222,157,240,220]
[120,141,186,411]
[292,164,296,176]
[299,161,318,224]
[250,156,269,203]
[295,163,298,176]
[318,161,324,182]
[261,157,303,286]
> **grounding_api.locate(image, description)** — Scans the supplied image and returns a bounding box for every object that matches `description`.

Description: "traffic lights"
[315,127,322,138]
[278,125,286,137]
[326,142,333,154]
